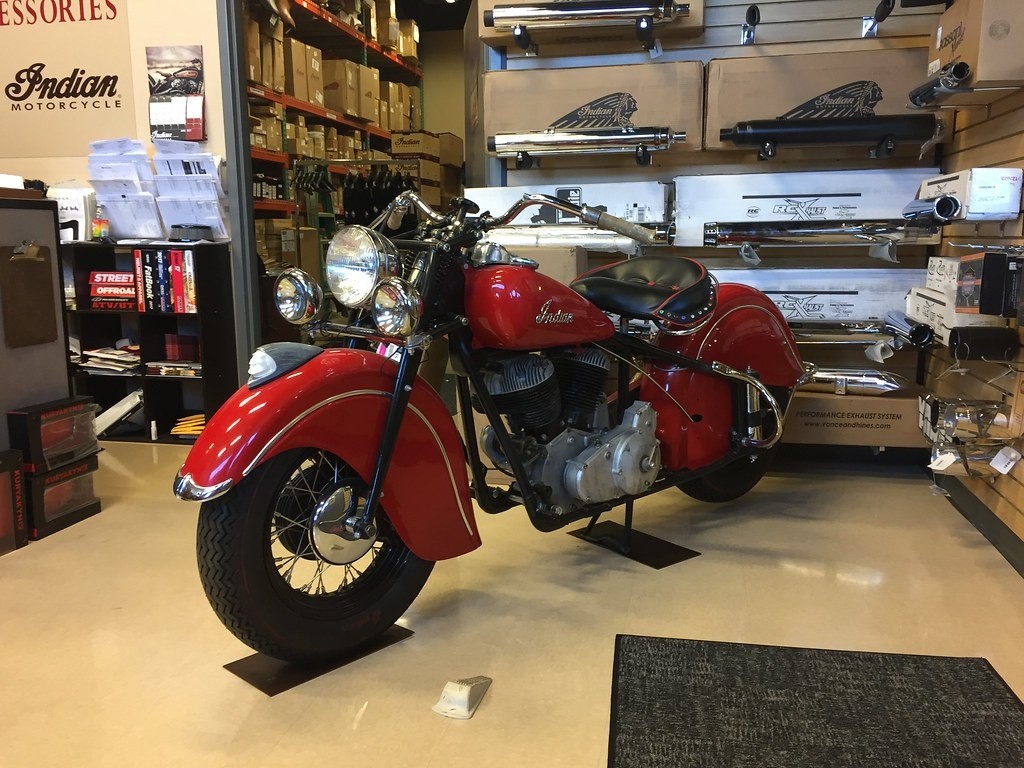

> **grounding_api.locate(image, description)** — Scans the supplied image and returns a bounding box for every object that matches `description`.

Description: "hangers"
[290,159,338,195]
[344,158,420,192]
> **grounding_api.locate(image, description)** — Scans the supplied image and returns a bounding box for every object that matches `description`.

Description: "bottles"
[92,202,110,243]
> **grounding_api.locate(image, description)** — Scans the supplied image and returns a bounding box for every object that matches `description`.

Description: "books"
[144,360,202,376]
[87,248,197,314]
[77,337,142,376]
[90,389,143,440]
[169,414,206,439]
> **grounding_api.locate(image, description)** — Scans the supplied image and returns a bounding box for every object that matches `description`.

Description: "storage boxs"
[242,0,324,108]
[0,395,106,558]
[904,252,1024,347]
[252,174,283,199]
[477,0,705,48]
[247,102,392,170]
[254,219,348,347]
[392,130,463,224]
[706,269,928,324]
[781,381,935,448]
[476,246,590,287]
[927,0,1024,107]
[921,168,1023,221]
[322,60,421,131]
[309,0,419,62]
[482,47,955,156]
[464,167,943,246]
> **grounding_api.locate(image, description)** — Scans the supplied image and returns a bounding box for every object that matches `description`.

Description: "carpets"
[607,634,1024,768]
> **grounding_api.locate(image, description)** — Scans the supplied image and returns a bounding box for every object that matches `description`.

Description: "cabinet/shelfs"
[60,238,239,445]
[240,0,425,215]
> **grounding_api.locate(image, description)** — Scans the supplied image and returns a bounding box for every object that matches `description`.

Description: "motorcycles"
[171,155,819,664]
[147,56,203,96]
